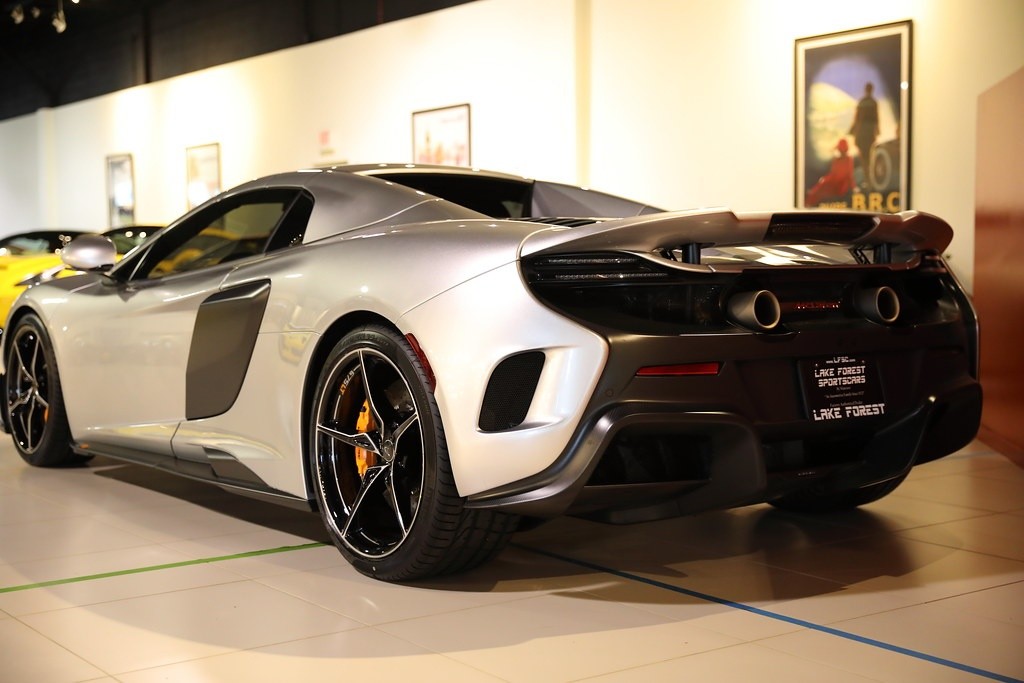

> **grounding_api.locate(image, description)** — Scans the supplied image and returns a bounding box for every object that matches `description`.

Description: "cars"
[0,219,244,344]
[5,164,984,584]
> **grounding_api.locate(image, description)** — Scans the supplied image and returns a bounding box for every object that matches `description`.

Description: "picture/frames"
[106,154,136,230]
[795,19,912,213]
[411,103,472,169]
[182,144,225,231]
